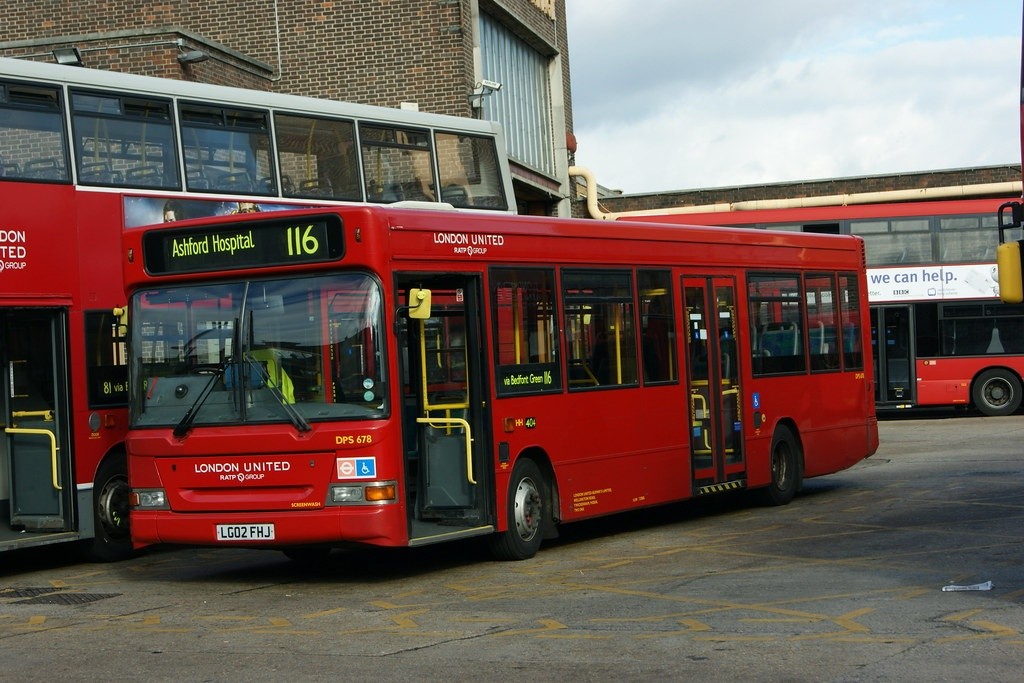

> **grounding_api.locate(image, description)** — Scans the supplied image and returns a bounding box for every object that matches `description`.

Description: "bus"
[122,192,883,566]
[614,197,1022,414]
[0,56,517,567]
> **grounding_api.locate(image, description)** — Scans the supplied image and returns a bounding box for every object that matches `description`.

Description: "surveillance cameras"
[482,79,502,92]
